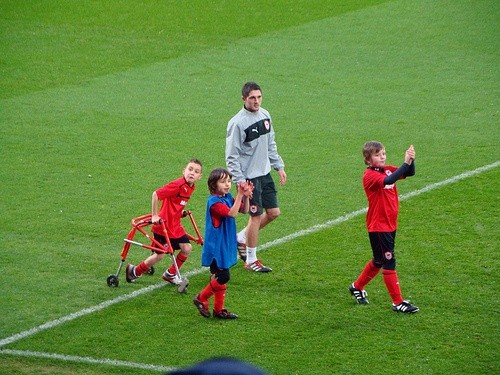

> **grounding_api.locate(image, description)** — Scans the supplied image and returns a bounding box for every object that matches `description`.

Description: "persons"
[126,158,202,285]
[192,168,254,320]
[226,82,288,273]
[348,141,419,313]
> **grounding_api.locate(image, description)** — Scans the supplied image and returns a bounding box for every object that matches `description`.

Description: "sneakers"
[348,283,369,303]
[193,294,211,317]
[125,263,142,283]
[213,308,238,319]
[245,259,272,272]
[162,269,181,284]
[392,300,419,313]
[237,240,247,262]
